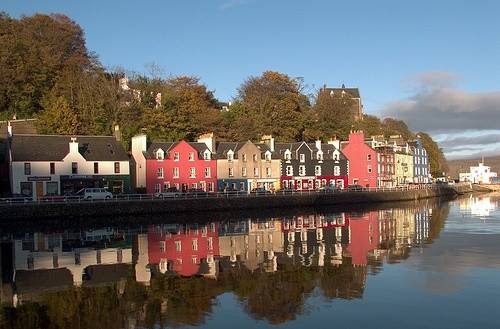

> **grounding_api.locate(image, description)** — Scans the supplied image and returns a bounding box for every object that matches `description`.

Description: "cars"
[276,187,297,193]
[37,192,66,202]
[0,193,33,203]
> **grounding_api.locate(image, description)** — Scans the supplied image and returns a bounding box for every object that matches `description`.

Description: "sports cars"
[154,189,182,198]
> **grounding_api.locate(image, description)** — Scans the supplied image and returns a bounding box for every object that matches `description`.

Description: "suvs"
[221,188,239,195]
[188,189,204,197]
[250,188,272,195]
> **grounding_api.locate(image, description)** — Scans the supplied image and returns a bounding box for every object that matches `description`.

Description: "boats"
[438,181,461,200]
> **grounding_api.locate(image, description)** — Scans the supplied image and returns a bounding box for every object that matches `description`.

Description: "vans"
[76,187,113,200]
